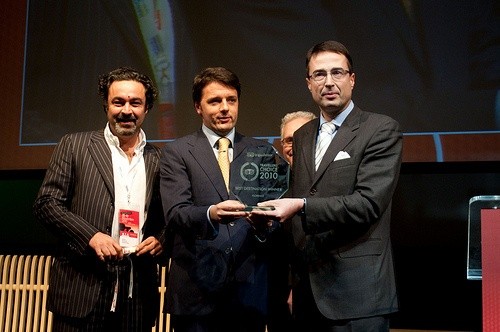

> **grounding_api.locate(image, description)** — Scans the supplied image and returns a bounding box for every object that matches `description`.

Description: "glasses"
[280,137,293,146]
[308,70,351,81]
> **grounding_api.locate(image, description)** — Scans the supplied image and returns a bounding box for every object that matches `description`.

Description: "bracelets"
[302,198,306,214]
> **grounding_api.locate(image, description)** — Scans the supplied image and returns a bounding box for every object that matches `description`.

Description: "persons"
[277,111,319,175]
[250,41,402,332]
[159,67,281,332]
[34,68,170,332]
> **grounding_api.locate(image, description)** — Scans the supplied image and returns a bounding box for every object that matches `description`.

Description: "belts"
[107,262,128,274]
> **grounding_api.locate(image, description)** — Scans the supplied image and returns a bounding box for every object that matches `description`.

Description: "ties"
[315,123,337,172]
[217,138,230,192]
